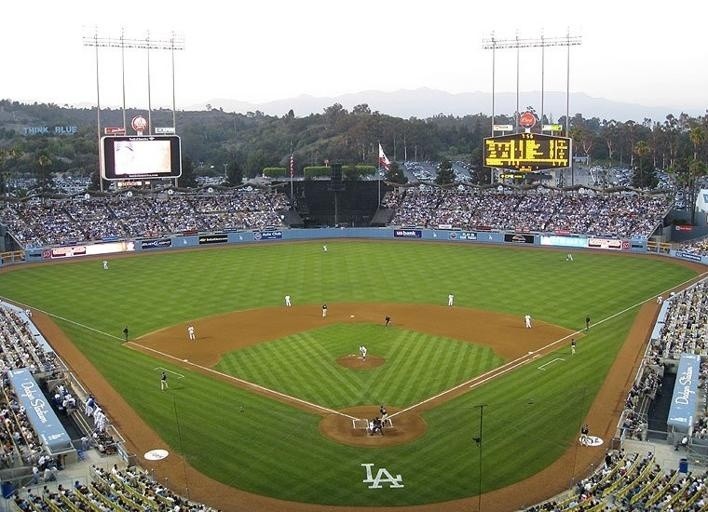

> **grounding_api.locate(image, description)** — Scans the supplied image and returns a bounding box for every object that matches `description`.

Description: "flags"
[379,144,392,171]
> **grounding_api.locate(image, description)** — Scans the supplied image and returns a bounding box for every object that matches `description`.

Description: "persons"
[321,244,329,256]
[103,259,109,269]
[384,314,392,328]
[285,295,292,307]
[360,344,367,361]
[374,190,708,261]
[321,303,328,320]
[368,406,393,435]
[0,194,292,249]
[521,279,708,512]
[448,293,455,311]
[0,300,223,511]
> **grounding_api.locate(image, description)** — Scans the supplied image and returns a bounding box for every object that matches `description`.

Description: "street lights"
[484,31,581,186]
[474,404,488,512]
[83,29,183,191]
